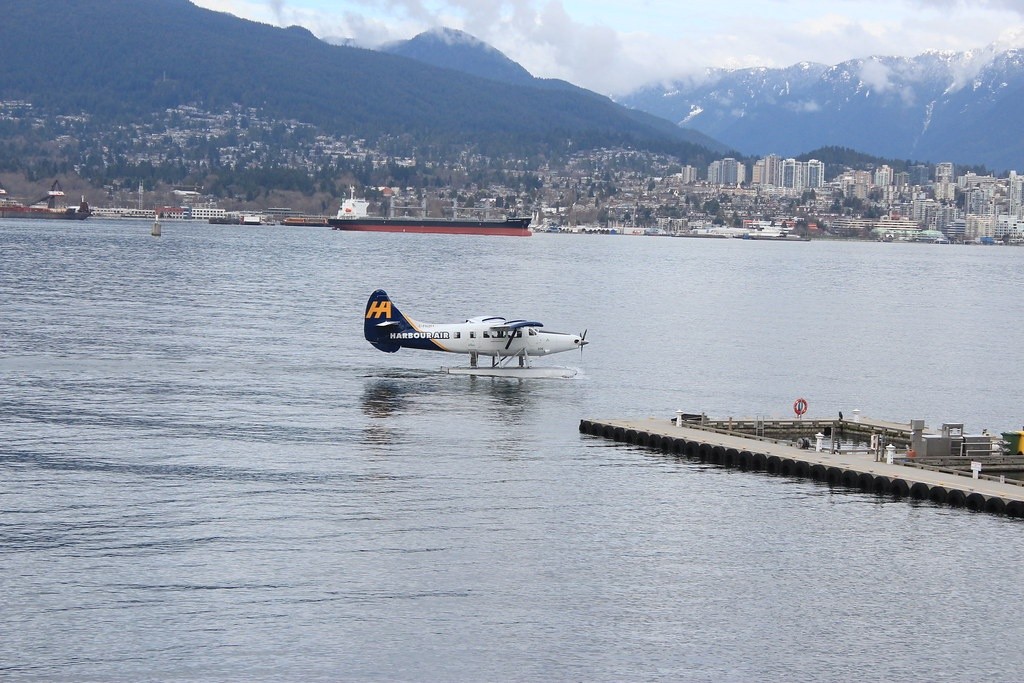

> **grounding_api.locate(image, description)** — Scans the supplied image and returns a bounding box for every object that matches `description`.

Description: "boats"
[329,186,533,236]
[209,217,239,224]
[0,204,91,220]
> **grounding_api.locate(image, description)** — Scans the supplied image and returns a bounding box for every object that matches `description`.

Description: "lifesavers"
[792,399,809,415]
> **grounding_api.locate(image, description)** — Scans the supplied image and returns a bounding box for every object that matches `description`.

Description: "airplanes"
[364,289,589,368]
